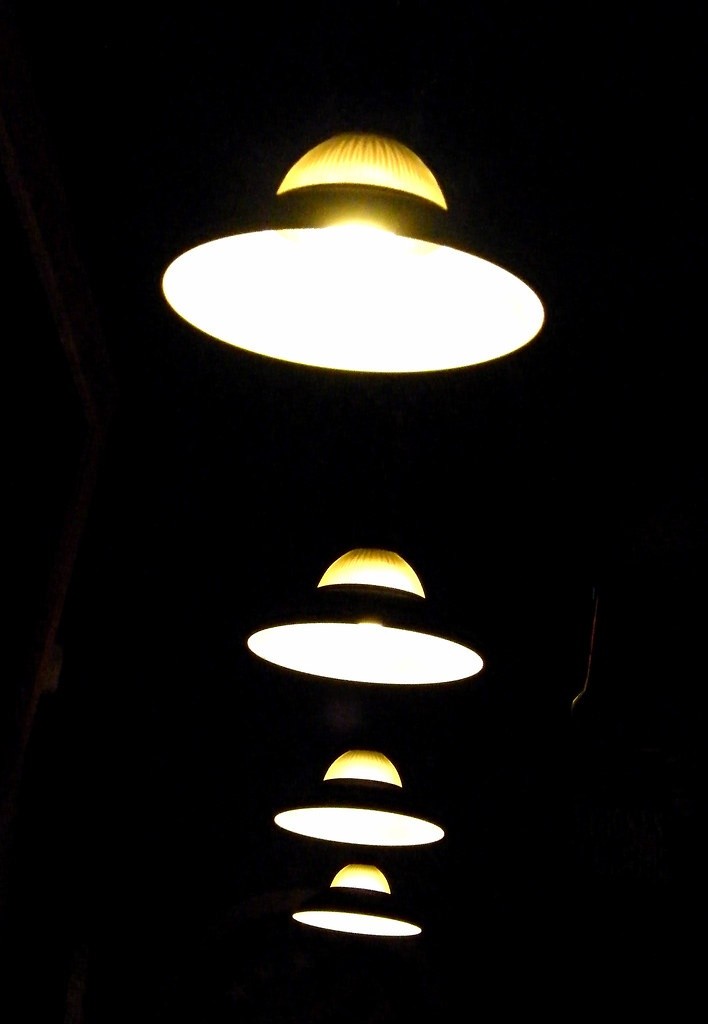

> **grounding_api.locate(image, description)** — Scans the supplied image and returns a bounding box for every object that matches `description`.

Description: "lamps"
[162,129,545,373]
[292,862,421,937]
[273,743,445,847]
[245,548,485,686]
